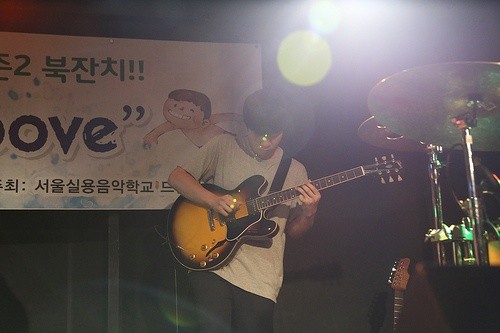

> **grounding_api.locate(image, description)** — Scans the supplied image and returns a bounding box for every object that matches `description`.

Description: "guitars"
[387,257,410,333]
[166,153,403,271]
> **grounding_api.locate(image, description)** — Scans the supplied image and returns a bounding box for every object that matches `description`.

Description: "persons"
[168,87,321,333]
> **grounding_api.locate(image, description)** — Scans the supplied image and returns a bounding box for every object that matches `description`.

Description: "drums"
[424,223,489,267]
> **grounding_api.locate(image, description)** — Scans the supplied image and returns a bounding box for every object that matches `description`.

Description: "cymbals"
[358,116,425,151]
[367,61,500,151]
